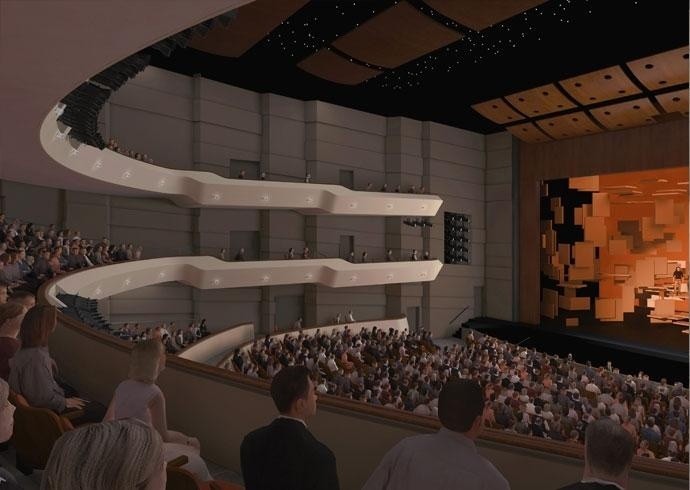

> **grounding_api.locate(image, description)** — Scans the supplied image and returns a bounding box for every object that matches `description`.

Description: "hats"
[37,416,164,490]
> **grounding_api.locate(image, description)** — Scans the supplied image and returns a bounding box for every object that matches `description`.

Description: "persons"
[561,417,635,489]
[672,266,684,297]
[230,309,689,463]
[101,338,215,483]
[106,138,155,165]
[236,366,341,488]
[117,314,211,358]
[2,215,169,488]
[363,380,512,488]
[219,242,431,265]
[238,170,427,194]
